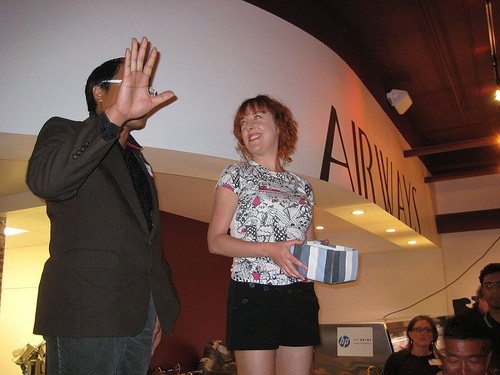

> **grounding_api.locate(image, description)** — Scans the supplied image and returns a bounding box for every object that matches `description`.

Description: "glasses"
[482,281,500,290]
[410,326,433,333]
[98,80,157,96]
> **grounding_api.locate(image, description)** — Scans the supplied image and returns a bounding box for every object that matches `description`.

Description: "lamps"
[386,89,413,115]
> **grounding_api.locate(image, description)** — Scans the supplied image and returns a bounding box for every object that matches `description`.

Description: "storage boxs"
[290,239,359,284]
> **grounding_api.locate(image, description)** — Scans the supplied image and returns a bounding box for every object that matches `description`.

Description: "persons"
[383,315,447,375]
[26,36,180,375]
[436,263,500,375]
[207,95,329,375]
